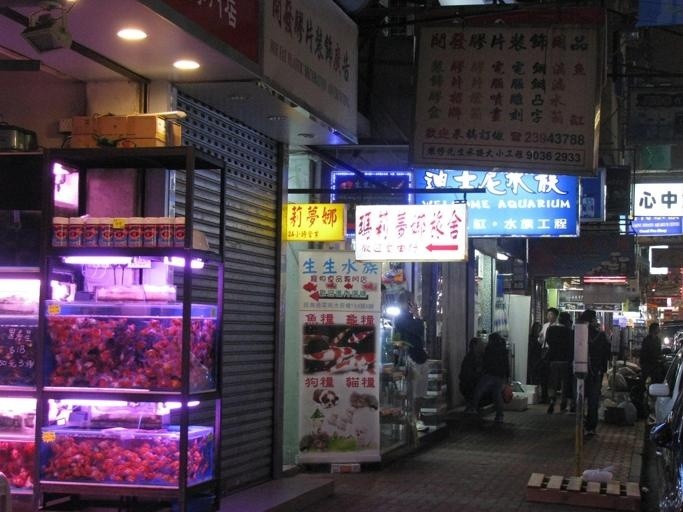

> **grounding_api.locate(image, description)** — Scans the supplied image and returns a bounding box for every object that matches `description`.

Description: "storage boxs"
[502,383,539,411]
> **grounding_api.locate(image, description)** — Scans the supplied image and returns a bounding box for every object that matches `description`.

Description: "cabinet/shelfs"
[30,143,233,512]
[1,142,80,508]
[336,349,448,473]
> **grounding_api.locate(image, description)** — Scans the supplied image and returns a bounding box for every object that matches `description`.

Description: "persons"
[637,322,663,387]
[457,337,493,407]
[391,289,433,433]
[381,282,388,309]
[526,307,612,439]
[470,331,510,423]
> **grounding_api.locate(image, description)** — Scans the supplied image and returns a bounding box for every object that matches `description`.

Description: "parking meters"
[572,322,588,475]
[609,323,619,403]
[622,326,633,368]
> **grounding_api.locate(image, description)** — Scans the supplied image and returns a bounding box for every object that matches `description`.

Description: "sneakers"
[584,428,596,436]
[463,407,479,415]
[495,415,504,423]
[416,420,429,432]
[547,402,576,413]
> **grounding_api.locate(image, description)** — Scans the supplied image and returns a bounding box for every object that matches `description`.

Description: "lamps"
[383,298,400,344]
[18,0,88,56]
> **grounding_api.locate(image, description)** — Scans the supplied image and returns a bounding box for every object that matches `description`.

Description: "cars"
[637,318,683,509]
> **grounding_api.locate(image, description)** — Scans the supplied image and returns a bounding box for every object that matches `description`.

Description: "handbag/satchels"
[550,360,569,379]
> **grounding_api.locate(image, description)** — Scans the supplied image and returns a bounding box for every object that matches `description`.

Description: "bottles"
[51,216,184,248]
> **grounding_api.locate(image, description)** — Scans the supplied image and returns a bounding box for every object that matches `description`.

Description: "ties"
[542,324,551,348]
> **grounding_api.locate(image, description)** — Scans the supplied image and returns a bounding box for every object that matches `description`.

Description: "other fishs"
[0,436,210,486]
[0,315,217,391]
